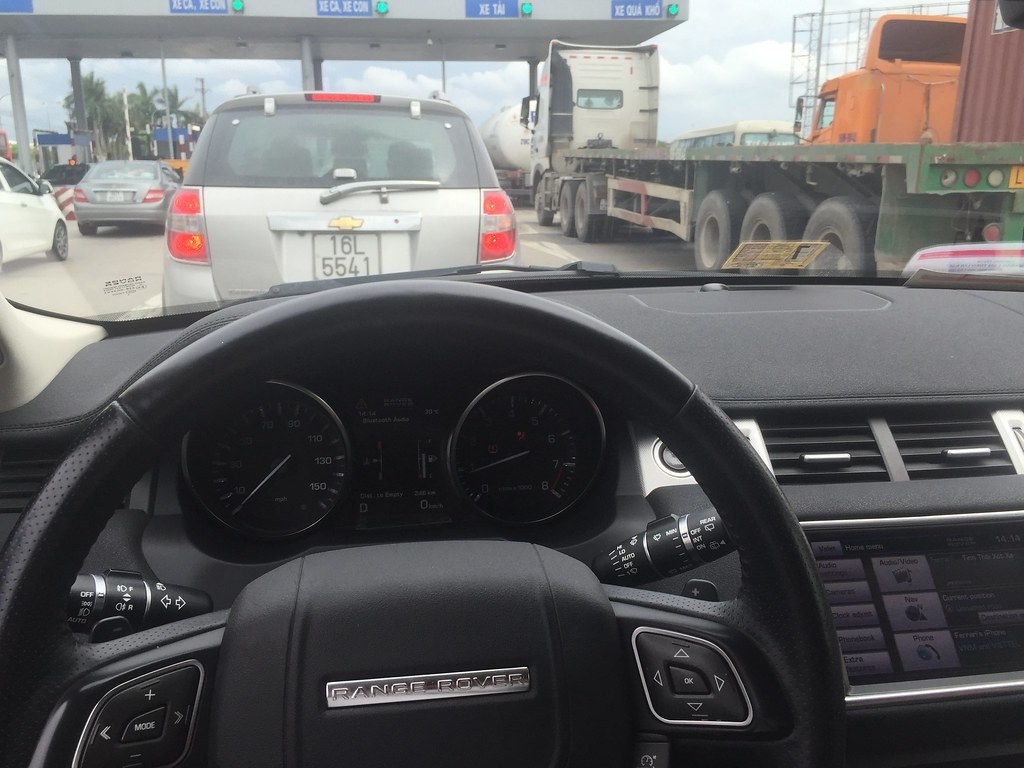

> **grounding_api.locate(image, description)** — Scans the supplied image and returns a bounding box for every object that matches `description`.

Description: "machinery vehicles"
[481,97,539,199]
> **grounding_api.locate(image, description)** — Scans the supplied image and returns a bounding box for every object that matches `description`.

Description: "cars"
[75,157,179,235]
[0,154,69,266]
[36,161,96,185]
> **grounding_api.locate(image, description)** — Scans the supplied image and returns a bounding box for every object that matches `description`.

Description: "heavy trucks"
[518,0,1024,244]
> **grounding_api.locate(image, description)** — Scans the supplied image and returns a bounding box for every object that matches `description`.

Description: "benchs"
[241,138,437,186]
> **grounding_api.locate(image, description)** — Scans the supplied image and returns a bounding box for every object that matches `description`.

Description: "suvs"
[160,83,520,312]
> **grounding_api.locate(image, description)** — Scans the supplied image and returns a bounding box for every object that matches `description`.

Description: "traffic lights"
[667,3,679,17]
[231,0,245,14]
[376,1,389,17]
[521,2,533,16]
